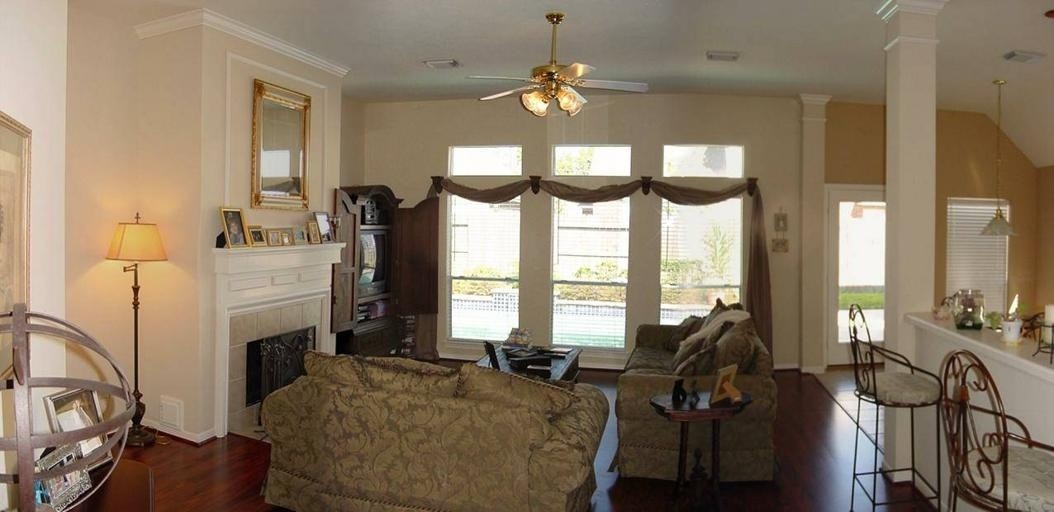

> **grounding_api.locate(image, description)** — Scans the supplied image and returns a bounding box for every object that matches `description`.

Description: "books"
[501,328,574,378]
[357,305,369,321]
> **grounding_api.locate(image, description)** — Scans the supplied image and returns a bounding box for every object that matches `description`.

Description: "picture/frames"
[312,212,336,244]
[215,207,251,249]
[39,388,113,472]
[0,110,33,384]
[248,225,268,247]
[36,442,94,511]
[266,229,282,246]
[708,363,742,405]
[292,224,309,245]
[251,78,312,211]
[306,220,322,244]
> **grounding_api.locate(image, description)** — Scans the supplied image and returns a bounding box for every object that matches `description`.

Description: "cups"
[1001,322,1023,343]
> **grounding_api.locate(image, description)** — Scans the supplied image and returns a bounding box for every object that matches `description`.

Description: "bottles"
[953,289,985,331]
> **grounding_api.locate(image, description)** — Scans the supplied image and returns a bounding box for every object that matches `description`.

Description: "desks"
[67,460,155,511]
[651,391,752,512]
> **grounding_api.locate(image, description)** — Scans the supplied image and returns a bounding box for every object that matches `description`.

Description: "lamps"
[979,79,1019,237]
[105,212,169,449]
[521,81,584,118]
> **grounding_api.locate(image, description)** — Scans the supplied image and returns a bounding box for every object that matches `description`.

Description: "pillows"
[453,364,580,420]
[355,355,461,399]
[302,348,370,390]
[667,297,756,375]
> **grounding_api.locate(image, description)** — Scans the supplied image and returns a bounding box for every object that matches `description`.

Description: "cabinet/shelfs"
[331,185,439,358]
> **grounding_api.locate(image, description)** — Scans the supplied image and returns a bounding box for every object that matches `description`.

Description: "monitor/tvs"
[357,230,388,296]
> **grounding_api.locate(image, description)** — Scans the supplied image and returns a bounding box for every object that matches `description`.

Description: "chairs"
[849,303,943,512]
[940,348,1054,511]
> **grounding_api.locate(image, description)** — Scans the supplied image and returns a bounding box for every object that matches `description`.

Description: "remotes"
[544,352,566,359]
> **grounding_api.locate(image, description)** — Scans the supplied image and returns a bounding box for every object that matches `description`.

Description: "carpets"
[813,368,885,454]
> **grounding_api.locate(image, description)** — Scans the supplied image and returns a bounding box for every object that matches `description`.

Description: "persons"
[226,215,246,245]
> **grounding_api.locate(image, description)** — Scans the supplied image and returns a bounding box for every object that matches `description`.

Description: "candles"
[1044,304,1054,345]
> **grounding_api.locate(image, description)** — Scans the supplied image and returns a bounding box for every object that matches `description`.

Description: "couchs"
[261,375,610,512]
[607,302,782,484]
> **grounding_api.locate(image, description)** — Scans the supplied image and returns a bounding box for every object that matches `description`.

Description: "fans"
[463,10,649,98]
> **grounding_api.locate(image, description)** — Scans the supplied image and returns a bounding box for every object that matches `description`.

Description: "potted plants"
[985,303,1029,342]
